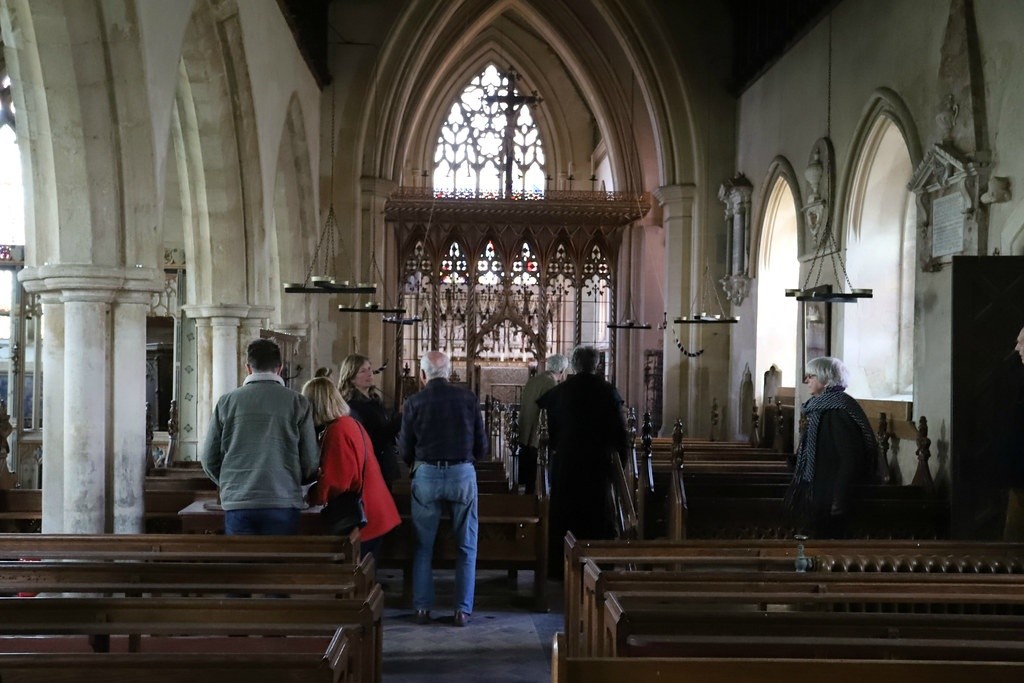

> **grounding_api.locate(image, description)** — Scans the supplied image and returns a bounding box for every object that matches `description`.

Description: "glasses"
[808,373,817,378]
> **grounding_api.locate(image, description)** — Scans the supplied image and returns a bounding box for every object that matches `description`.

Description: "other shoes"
[456,610,470,625]
[415,609,429,625]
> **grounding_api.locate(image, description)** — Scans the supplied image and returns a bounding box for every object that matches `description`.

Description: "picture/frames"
[801,285,834,384]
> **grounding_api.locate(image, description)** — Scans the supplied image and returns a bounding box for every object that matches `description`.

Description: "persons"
[1004,327,1024,543]
[314,367,335,382]
[398,351,488,627]
[336,353,402,495]
[776,356,886,540]
[200,338,321,637]
[516,344,630,588]
[301,377,401,591]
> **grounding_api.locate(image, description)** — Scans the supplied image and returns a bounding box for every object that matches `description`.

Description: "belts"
[426,458,473,466]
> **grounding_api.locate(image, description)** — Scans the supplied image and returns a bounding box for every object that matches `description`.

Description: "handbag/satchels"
[319,496,368,534]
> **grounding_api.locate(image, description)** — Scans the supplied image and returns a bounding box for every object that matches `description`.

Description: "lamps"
[607,72,653,330]
[787,1,873,304]
[284,78,380,296]
[337,94,407,315]
[675,74,739,326]
[382,137,423,327]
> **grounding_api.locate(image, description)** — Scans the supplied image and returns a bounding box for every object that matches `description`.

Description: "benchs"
[0,408,1023,683]
